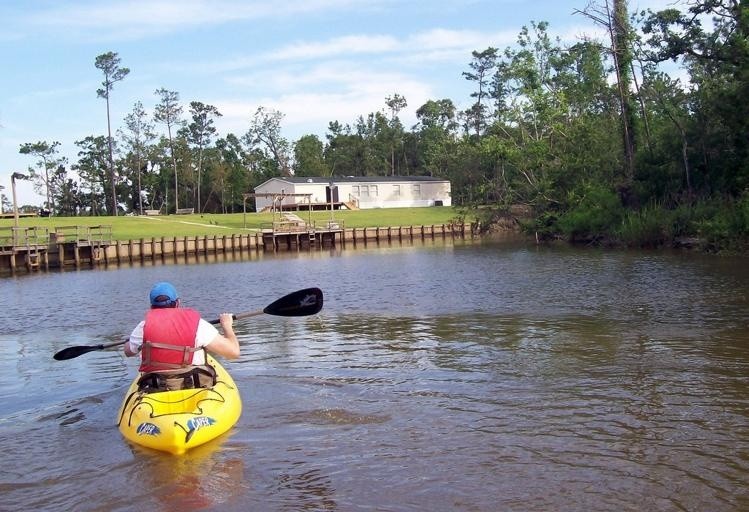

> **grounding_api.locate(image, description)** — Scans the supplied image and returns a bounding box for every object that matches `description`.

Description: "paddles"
[54,288,323,360]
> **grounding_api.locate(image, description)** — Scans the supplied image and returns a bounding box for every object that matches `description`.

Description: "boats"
[117,351,245,455]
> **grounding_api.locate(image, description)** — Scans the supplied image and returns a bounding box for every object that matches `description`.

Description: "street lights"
[11,171,26,247]
[327,178,336,222]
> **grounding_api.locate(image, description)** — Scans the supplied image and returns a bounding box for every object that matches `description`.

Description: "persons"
[123,280,241,390]
[140,455,243,512]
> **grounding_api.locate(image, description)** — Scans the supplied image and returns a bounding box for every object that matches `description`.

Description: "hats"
[149,282,178,306]
